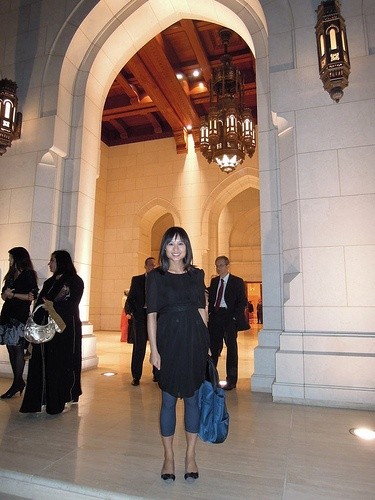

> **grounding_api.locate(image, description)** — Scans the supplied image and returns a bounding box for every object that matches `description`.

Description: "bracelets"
[13,293,15,297]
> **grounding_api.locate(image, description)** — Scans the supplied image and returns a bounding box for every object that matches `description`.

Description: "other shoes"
[131,377,139,386]
[184,472,198,481]
[221,382,236,391]
[160,473,175,482]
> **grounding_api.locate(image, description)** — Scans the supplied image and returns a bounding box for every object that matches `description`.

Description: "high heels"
[0,380,26,399]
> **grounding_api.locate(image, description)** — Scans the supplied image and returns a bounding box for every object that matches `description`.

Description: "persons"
[207,256,251,390]
[248,301,254,324]
[0,247,39,399]
[125,257,160,386]
[19,250,84,416]
[122,289,129,308]
[145,227,212,481]
[257,299,263,324]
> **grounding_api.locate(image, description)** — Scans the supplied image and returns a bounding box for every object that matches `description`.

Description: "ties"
[214,279,224,312]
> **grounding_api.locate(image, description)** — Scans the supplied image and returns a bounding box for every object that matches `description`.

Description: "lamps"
[200,28,256,175]
[0,77,23,155]
[314,0,352,104]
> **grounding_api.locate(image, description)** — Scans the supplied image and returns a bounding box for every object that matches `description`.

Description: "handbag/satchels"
[198,355,230,444]
[23,304,56,343]
